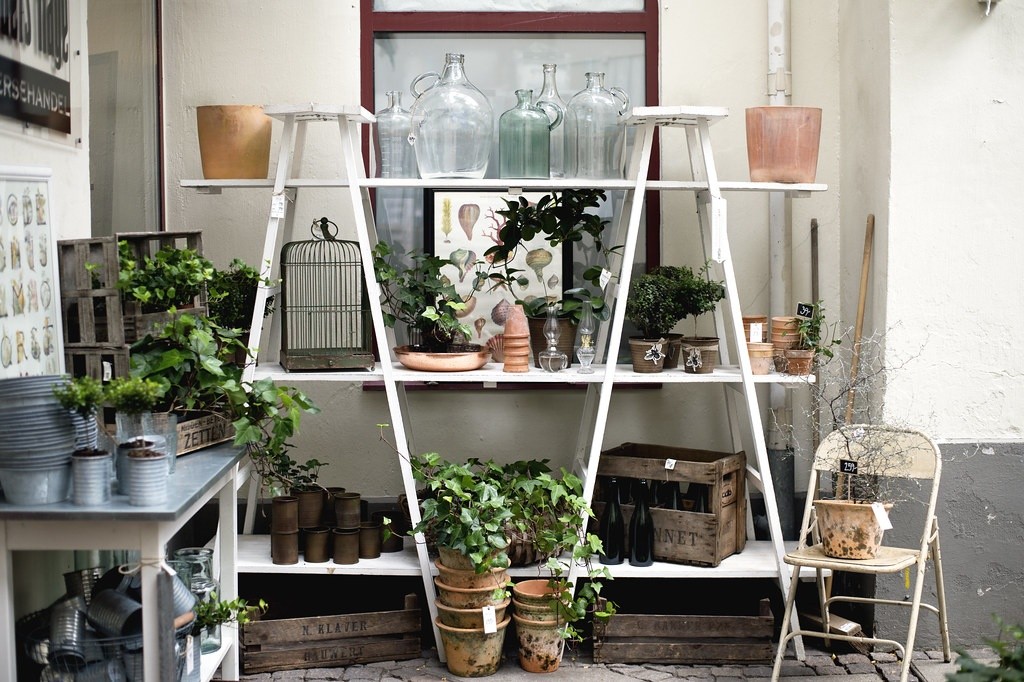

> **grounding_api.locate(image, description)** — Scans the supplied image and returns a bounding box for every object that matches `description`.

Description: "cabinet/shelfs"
[0,444,248,682]
[179,105,834,651]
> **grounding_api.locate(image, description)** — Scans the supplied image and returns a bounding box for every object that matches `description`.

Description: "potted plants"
[681,260,725,374]
[483,189,610,367]
[134,315,318,493]
[656,265,684,369]
[296,458,329,529]
[628,273,668,372]
[785,301,843,376]
[113,376,155,495]
[128,378,170,507]
[87,241,212,343]
[51,374,112,506]
[499,458,617,670]
[205,260,282,366]
[373,422,510,600]
[372,242,486,371]
[792,321,981,561]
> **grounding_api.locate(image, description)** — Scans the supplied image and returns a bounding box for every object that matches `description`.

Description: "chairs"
[772,425,950,682]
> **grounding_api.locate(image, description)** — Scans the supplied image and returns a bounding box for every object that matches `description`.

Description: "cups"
[29,545,205,681]
[268,484,404,565]
[70,412,179,508]
[502,305,531,373]
[742,314,801,375]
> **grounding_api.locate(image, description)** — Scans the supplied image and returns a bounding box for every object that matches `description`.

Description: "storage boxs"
[55,230,236,459]
[587,581,773,665]
[593,443,748,568]
[238,584,421,674]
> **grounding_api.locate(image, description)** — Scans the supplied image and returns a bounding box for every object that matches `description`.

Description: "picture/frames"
[424,189,575,359]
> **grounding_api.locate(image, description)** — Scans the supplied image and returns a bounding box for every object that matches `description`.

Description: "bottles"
[376,52,635,180]
[597,477,627,565]
[628,478,654,567]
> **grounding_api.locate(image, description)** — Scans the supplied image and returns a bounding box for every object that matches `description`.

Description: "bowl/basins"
[0,372,101,507]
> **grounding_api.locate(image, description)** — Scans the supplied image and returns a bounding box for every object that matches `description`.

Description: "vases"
[433,558,570,678]
[197,104,271,179]
[743,314,800,374]
[502,305,531,373]
[745,106,823,184]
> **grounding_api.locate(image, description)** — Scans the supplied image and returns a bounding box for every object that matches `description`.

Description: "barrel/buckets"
[512,580,572,673]
